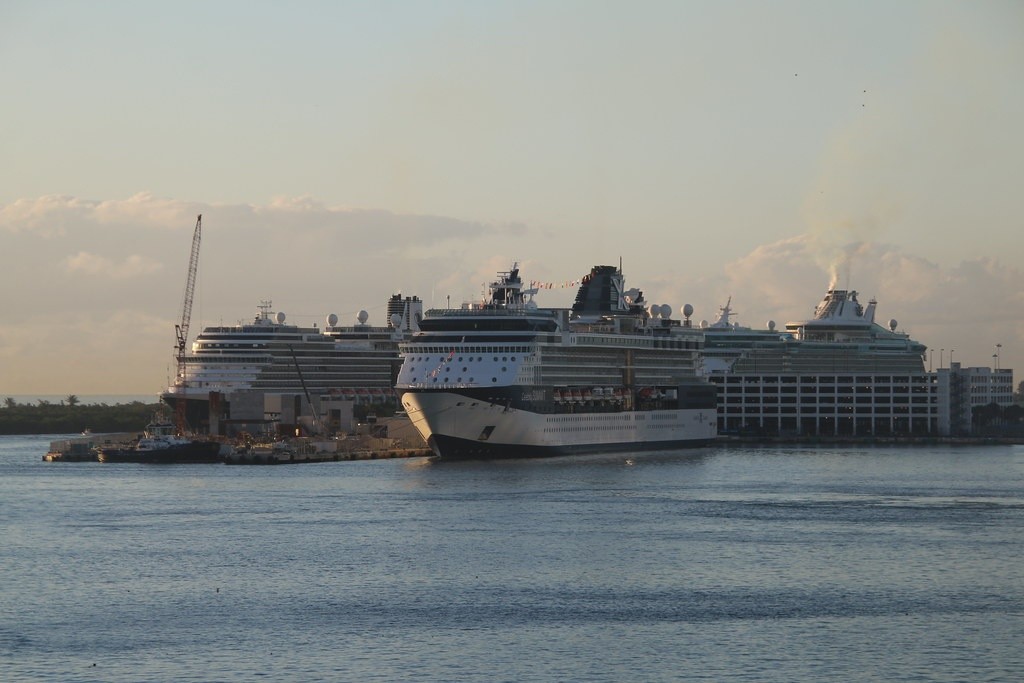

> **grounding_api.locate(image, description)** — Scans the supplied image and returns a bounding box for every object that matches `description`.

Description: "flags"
[582,271,596,283]
[531,278,580,289]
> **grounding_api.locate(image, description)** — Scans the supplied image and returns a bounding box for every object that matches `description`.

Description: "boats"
[702,300,785,373]
[92,410,220,464]
[730,287,927,374]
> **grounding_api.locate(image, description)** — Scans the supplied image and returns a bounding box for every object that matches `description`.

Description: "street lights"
[950,349,955,365]
[992,343,1001,371]
[930,350,934,371]
[940,349,944,367]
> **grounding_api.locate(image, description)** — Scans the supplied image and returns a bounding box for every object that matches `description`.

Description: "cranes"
[173,216,205,431]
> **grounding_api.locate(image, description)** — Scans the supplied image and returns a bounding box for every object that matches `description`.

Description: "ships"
[161,299,418,434]
[392,264,719,461]
